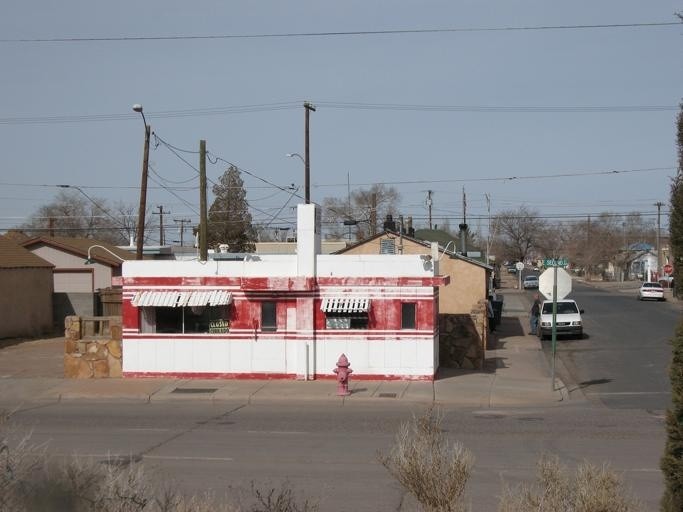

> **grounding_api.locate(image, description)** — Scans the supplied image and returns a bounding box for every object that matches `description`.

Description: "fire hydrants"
[332,355,353,396]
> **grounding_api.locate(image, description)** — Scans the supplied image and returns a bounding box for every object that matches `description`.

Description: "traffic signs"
[544,258,568,267]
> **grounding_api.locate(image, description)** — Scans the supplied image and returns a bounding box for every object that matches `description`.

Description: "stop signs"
[665,265,672,273]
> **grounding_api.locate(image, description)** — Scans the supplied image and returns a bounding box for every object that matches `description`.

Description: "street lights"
[286,152,310,204]
[132,103,150,260]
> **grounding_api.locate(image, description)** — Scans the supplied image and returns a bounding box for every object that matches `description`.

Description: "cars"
[507,266,517,274]
[638,282,664,301]
[658,273,674,284]
[523,275,539,289]
[537,299,584,340]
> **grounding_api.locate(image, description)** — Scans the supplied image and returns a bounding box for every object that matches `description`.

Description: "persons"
[486,295,497,335]
[527,291,541,335]
